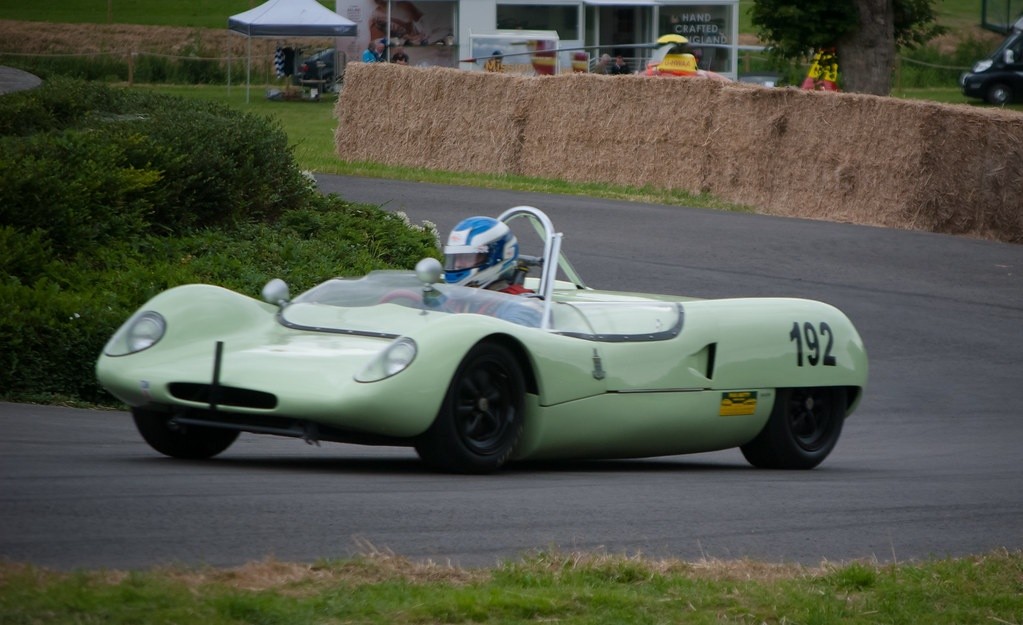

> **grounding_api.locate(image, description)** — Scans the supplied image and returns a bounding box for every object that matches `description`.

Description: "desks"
[302,80,326,99]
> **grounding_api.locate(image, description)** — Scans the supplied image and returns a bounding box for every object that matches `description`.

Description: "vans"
[959,12,1022,108]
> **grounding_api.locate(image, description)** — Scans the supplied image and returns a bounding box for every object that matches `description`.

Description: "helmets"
[443,215,520,298]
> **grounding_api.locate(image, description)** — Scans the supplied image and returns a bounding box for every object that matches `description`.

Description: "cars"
[96,206,868,477]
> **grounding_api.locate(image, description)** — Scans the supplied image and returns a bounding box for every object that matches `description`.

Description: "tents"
[227,0,358,105]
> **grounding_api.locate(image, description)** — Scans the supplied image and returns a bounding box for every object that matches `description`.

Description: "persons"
[373,43,386,62]
[437,216,554,328]
[595,54,612,74]
[361,42,377,63]
[390,45,409,65]
[610,56,631,74]
